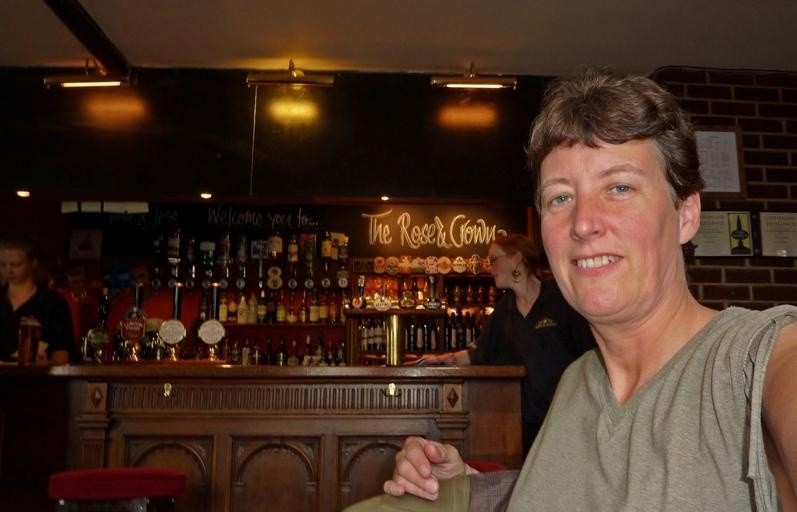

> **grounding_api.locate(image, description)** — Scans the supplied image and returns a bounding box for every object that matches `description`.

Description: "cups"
[16,319,42,365]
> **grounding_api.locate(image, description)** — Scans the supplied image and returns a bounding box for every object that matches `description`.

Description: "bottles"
[355,272,497,315]
[222,331,348,369]
[217,288,347,324]
[353,315,485,354]
[153,224,348,278]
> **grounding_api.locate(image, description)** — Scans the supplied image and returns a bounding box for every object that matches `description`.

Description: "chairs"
[48,469,187,512]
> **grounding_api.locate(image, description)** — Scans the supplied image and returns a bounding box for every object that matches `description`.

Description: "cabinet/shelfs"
[45,362,528,512]
[346,271,499,367]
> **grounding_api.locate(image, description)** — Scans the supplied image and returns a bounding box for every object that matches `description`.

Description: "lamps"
[430,62,518,93]
[42,54,136,89]
[246,59,335,89]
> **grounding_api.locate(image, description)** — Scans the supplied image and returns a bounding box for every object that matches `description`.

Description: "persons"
[0,239,77,365]
[378,61,796,510]
[403,233,596,469]
